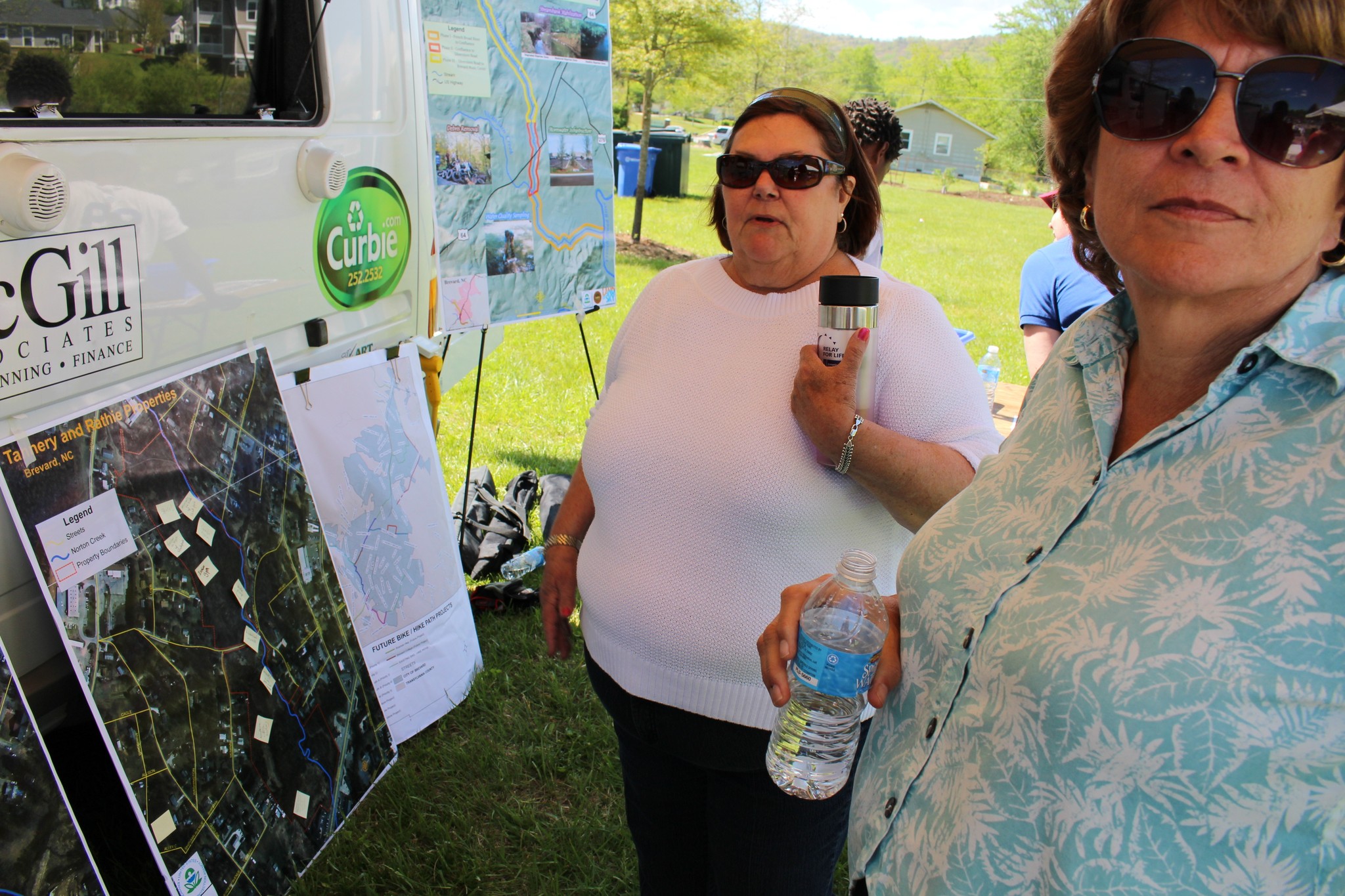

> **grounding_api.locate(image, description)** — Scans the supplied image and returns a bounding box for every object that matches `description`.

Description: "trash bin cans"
[615,142,662,197]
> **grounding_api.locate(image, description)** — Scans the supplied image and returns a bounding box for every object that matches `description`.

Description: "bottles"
[501,546,545,581]
[978,345,1001,412]
[766,548,889,800]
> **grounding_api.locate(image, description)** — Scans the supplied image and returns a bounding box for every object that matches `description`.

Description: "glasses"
[717,154,845,189]
[1089,38,1345,169]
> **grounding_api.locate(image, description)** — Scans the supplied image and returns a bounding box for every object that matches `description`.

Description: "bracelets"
[543,533,582,556]
[836,414,864,474]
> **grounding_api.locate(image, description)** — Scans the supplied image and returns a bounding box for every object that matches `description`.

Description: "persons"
[542,0,1345,896]
[505,229,514,254]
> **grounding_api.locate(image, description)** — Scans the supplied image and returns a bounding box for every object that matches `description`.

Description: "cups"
[819,273,880,421]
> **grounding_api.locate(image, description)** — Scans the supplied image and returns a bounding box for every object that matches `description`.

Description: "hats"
[1039,188,1060,215]
